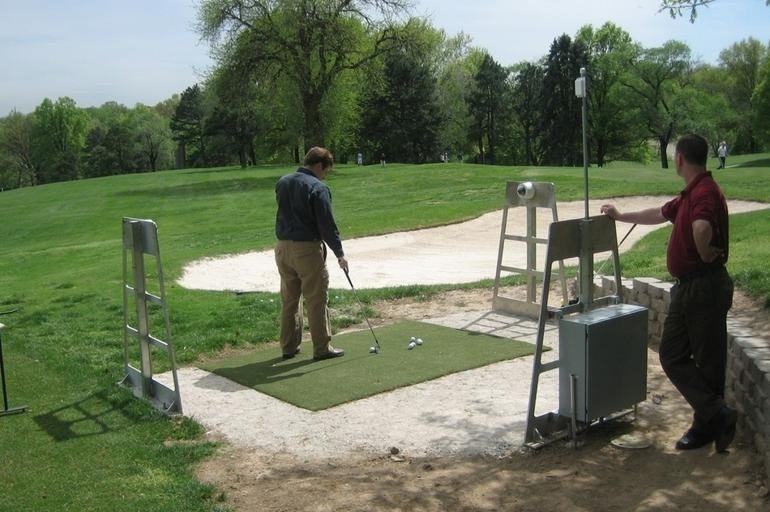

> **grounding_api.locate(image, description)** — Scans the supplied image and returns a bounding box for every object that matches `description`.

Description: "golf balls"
[369,347,376,353]
[560,299,564,305]
[408,337,422,349]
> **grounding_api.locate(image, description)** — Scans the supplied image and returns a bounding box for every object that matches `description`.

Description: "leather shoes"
[675,429,715,449]
[282,346,300,358]
[314,349,344,360]
[714,406,737,453]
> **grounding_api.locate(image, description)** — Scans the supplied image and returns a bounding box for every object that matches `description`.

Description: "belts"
[673,264,726,285]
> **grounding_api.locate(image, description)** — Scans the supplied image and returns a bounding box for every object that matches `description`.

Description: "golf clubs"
[560,223,637,307]
[338,260,380,354]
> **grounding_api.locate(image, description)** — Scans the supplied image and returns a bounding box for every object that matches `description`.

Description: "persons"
[716,140,730,169]
[599,131,740,454]
[273,144,350,362]
[379,152,387,168]
[456,151,464,163]
[597,142,605,168]
[440,153,444,163]
[357,150,363,166]
[443,152,449,163]
